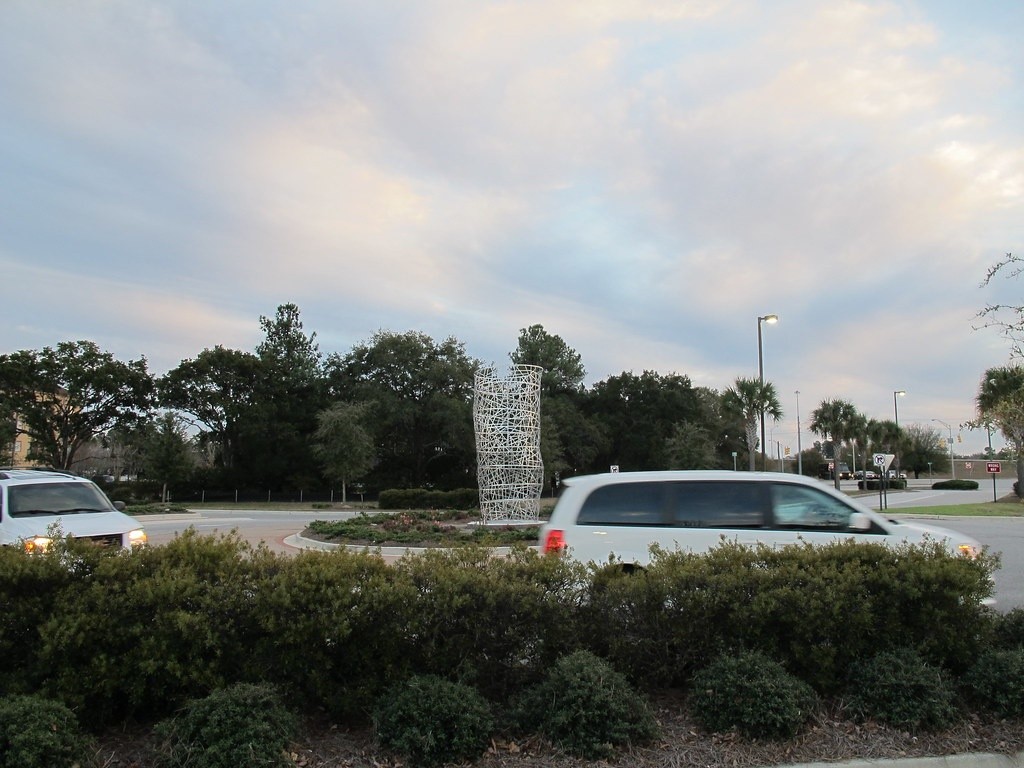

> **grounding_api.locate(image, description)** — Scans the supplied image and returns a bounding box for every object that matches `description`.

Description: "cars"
[885,470,907,479]
[852,470,875,480]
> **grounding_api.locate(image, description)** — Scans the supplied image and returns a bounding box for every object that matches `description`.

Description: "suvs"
[540,469,996,611]
[0,467,148,579]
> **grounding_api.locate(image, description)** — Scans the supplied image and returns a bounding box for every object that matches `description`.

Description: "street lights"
[932,418,954,477]
[894,391,906,475]
[794,390,802,475]
[758,314,778,471]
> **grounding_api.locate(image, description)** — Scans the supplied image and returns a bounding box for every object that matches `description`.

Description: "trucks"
[818,462,851,480]
